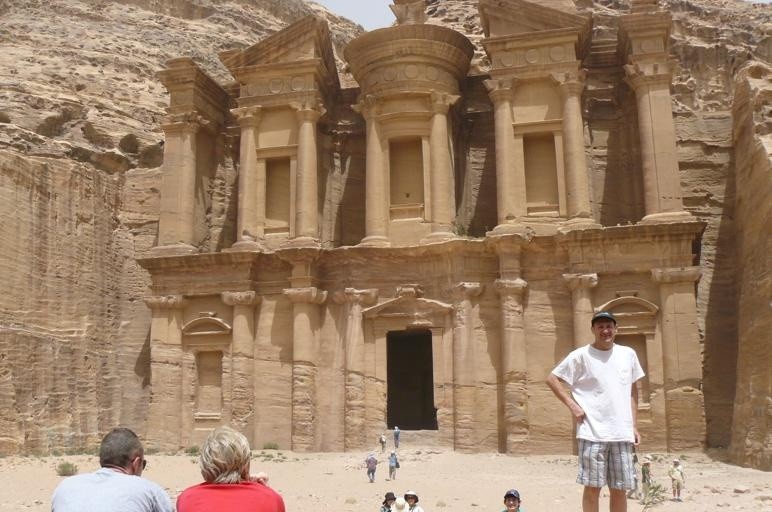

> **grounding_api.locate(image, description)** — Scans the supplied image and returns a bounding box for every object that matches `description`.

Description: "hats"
[385,493,396,500]
[404,491,418,503]
[505,490,519,498]
[591,310,617,325]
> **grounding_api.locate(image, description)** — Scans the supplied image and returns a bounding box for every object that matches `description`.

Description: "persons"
[393,426,400,449]
[176,426,286,512]
[380,433,387,453]
[626,454,685,501]
[545,311,646,512]
[388,452,397,480]
[50,428,176,512]
[366,453,377,482]
[380,492,396,512]
[401,490,424,512]
[500,489,525,512]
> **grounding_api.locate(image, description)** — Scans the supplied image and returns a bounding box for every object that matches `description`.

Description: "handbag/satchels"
[396,462,399,468]
